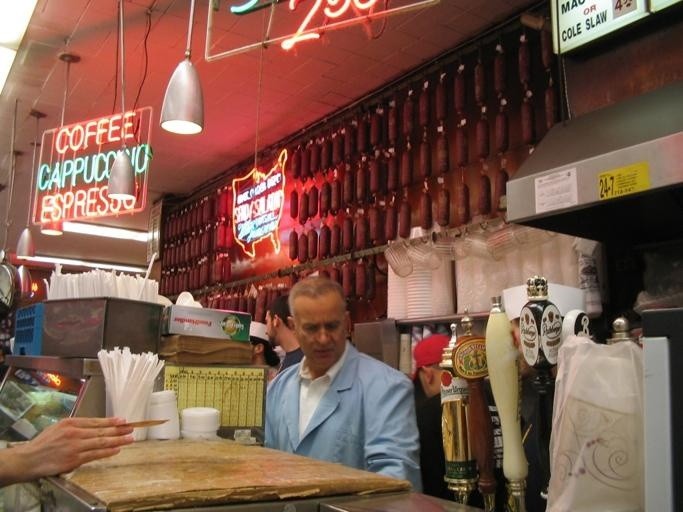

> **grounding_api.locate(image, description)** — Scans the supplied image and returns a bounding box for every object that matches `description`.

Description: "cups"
[105,379,155,441]
[386,227,432,321]
[383,219,556,279]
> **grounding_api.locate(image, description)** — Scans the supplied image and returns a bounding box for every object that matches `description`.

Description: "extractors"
[504,81,682,225]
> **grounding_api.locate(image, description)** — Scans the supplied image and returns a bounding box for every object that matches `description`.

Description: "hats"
[250,321,269,341]
[411,334,449,381]
[503,283,583,322]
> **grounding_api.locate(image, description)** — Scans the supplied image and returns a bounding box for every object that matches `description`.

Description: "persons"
[249,321,280,430]
[411,333,506,511]
[0,414,135,489]
[263,277,424,493]
[265,294,304,374]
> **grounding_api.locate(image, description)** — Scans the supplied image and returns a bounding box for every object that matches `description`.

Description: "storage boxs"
[157,305,252,339]
[41,297,164,358]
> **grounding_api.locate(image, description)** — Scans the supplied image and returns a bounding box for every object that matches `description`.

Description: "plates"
[181,406,222,441]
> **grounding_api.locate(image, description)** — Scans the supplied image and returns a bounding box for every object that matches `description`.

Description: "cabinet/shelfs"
[355,314,499,390]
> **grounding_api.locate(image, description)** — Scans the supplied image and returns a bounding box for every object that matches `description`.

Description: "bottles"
[149,391,179,439]
[398,333,411,373]
[422,324,435,338]
[410,325,422,374]
[577,254,604,319]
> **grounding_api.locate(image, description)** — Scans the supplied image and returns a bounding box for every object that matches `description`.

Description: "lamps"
[16,111,48,259]
[105,1,139,201]
[157,0,204,136]
[12,266,33,297]
[39,51,81,236]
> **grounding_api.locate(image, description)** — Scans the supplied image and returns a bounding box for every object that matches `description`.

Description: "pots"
[0,259,20,320]
